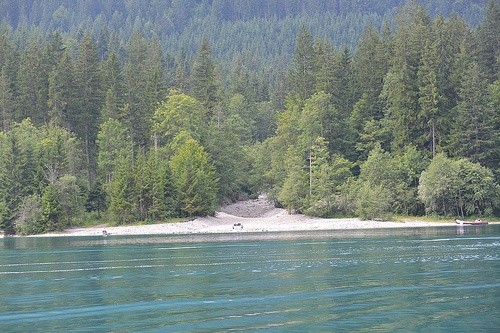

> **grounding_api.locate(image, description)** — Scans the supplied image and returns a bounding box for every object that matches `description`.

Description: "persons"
[474,219,482,223]
